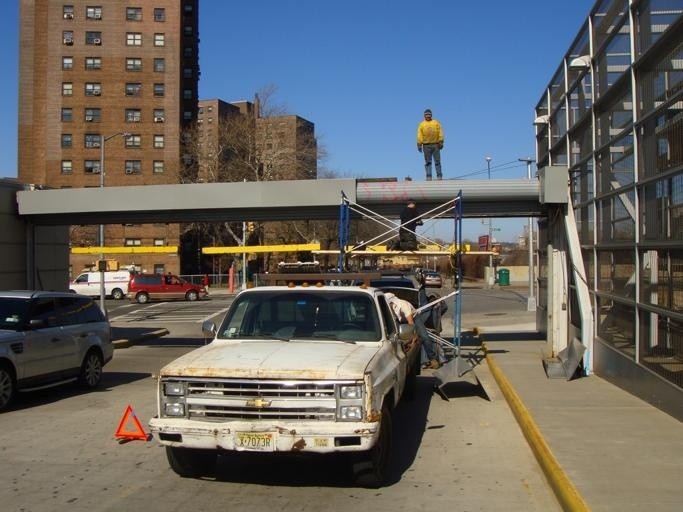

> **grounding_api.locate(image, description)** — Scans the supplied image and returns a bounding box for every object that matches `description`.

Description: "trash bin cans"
[498,269,509,286]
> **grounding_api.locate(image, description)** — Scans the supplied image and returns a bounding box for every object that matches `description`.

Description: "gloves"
[418,146,422,152]
[439,141,443,149]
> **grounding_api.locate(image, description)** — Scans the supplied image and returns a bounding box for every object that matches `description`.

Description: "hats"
[407,199,416,205]
[424,109,431,113]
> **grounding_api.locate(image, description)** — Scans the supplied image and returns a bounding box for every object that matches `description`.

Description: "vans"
[68,265,135,300]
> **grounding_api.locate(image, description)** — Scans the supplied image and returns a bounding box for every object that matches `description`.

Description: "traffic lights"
[97,259,118,271]
[249,222,254,232]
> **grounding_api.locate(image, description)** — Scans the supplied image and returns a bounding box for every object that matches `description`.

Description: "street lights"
[486,157,491,286]
[100,131,132,321]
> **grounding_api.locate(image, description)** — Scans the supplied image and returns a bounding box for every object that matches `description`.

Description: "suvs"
[0,290,112,410]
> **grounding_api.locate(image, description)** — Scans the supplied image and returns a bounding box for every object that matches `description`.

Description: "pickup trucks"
[148,282,420,478]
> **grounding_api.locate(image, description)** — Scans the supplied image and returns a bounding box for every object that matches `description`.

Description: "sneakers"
[387,240,400,251]
[431,359,439,369]
[427,176,442,181]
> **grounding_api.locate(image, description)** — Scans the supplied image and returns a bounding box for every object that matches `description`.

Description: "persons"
[417,109,444,181]
[399,198,424,249]
[419,293,447,369]
[201,274,211,297]
[166,272,172,283]
[384,291,439,370]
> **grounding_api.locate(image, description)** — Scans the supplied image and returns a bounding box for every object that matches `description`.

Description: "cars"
[356,268,442,309]
[127,272,208,303]
